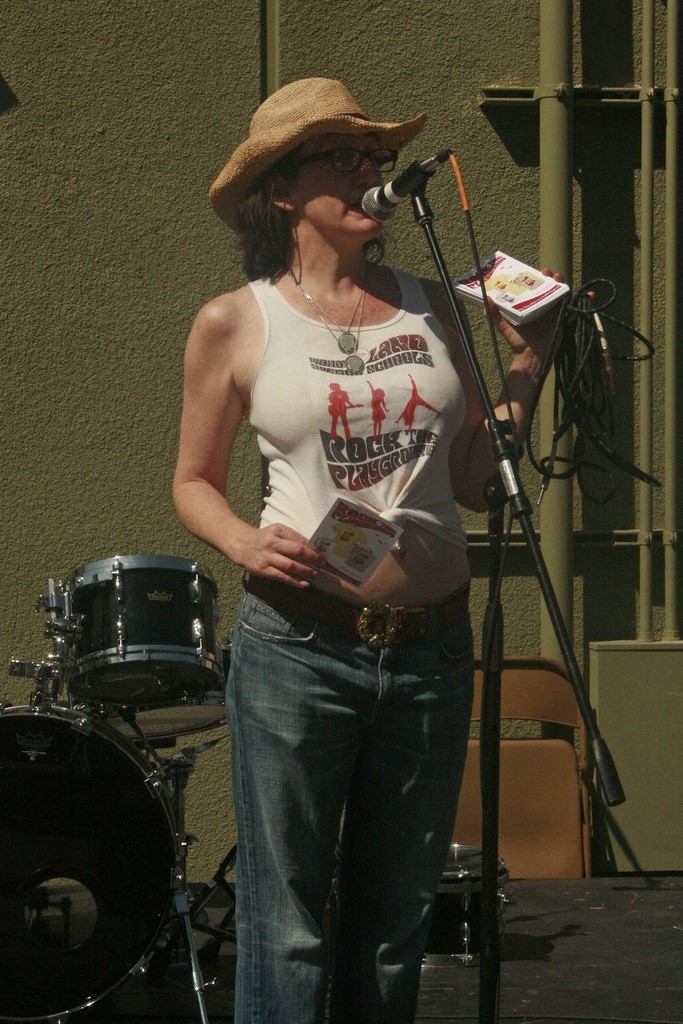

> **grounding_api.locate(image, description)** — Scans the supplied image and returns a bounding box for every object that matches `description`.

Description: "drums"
[421,844,509,968]
[0,705,178,1024]
[49,553,230,737]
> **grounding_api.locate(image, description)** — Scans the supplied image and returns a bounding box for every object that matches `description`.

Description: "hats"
[209,77,427,233]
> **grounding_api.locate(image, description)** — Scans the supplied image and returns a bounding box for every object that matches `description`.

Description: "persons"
[170,75,559,1024]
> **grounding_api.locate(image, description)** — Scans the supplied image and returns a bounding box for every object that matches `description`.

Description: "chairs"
[446,658,593,877]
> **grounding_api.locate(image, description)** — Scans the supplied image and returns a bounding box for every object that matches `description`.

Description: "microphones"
[361,148,455,221]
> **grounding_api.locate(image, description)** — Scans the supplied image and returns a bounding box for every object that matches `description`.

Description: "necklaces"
[284,265,366,374]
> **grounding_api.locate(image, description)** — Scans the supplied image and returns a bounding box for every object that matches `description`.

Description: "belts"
[247,574,470,648]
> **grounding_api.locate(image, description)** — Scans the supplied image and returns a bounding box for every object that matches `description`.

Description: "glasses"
[294,148,399,173]
